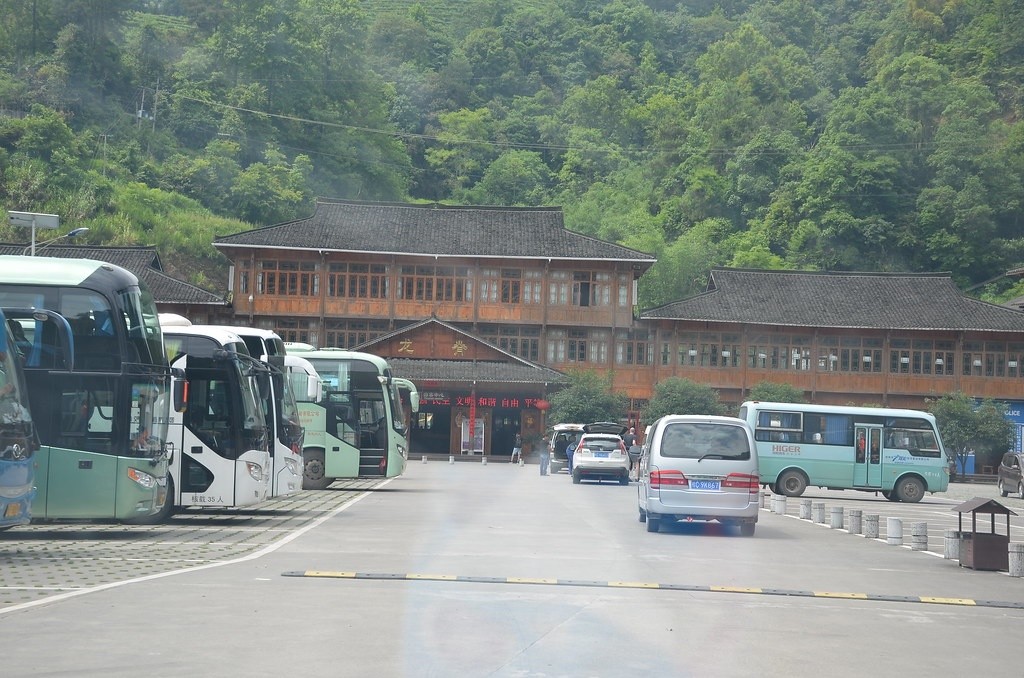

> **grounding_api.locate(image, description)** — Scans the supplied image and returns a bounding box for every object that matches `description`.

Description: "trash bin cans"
[951,496,1019,573]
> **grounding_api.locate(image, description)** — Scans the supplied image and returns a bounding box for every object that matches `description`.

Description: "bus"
[192,325,322,499]
[0,254,188,518]
[12,314,284,525]
[738,401,950,503]
[284,342,419,489]
[0,306,75,531]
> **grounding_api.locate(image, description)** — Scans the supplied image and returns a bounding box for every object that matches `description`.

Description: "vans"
[638,414,760,536]
[550,423,588,474]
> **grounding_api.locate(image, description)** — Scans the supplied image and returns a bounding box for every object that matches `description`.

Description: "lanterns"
[536,400,550,414]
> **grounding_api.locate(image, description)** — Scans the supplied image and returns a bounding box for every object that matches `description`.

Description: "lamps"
[688,344,1019,368]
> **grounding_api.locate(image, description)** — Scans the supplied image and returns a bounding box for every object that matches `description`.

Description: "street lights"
[23,227,89,256]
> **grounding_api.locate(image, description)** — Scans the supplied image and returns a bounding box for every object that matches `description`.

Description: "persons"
[539,434,551,476]
[509,433,522,463]
[623,427,637,472]
[566,442,577,476]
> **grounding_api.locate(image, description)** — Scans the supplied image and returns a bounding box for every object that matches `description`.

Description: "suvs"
[572,432,630,485]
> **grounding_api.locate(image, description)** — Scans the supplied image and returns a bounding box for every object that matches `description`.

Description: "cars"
[997,452,1024,499]
